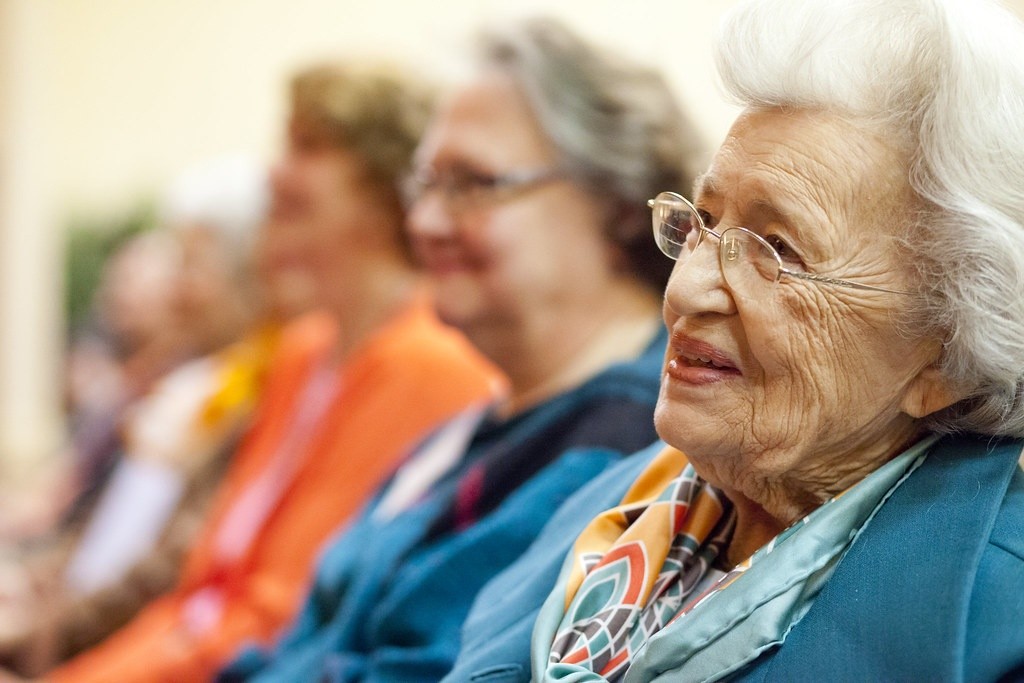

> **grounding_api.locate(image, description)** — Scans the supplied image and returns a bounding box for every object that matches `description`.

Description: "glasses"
[646,191,917,297]
[404,166,564,212]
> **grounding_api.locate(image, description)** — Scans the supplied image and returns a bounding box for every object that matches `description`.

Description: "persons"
[212,19,715,683]
[1,63,510,683]
[444,0,1024,683]
